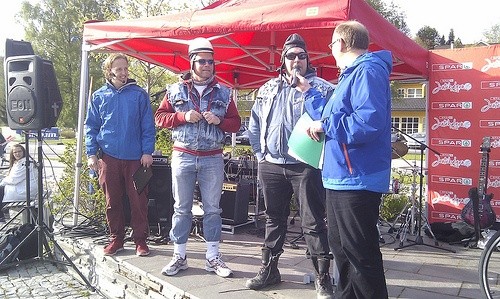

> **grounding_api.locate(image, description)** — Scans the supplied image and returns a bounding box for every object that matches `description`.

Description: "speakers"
[5,53,63,130]
[122,163,174,227]
[217,180,250,224]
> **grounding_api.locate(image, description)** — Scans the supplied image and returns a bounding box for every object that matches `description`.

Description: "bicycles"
[478,228,500,299]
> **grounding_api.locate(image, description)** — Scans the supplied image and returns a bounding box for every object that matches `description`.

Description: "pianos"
[223,159,267,216]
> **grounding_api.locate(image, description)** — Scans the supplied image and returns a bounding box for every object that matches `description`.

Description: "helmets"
[188,37,214,57]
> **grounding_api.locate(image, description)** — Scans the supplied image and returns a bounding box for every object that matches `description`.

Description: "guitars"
[460,136,497,230]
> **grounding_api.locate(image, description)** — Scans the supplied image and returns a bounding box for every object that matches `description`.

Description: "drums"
[383,178,400,194]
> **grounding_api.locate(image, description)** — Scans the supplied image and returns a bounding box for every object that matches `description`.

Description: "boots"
[307,254,334,299]
[245,248,284,289]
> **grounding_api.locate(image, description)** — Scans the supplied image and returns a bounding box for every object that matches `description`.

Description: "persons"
[153,38,241,278]
[290,20,393,299]
[0,144,37,223]
[245,34,333,299]
[85,52,156,256]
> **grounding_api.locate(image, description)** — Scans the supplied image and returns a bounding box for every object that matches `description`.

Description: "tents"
[74,0,431,225]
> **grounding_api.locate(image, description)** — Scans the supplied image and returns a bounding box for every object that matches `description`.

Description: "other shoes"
[3,212,10,219]
[0,217,6,224]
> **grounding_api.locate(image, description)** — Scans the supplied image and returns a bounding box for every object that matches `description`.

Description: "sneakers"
[104,239,124,256]
[161,252,188,276]
[135,239,150,256]
[205,252,234,278]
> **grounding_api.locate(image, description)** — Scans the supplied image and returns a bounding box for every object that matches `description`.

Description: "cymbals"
[390,134,410,159]
[398,166,429,170]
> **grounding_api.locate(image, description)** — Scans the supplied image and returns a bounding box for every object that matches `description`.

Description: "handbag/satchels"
[424,221,475,243]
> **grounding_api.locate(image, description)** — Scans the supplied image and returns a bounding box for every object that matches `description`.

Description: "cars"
[223,125,246,148]
[390,131,408,152]
[236,129,250,147]
[406,132,427,148]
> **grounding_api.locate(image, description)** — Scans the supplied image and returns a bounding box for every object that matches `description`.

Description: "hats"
[279,33,310,92]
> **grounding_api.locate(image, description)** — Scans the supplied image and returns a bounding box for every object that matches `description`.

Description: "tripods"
[376,127,456,254]
[0,127,95,291]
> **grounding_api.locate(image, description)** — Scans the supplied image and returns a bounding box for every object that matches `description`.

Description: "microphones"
[291,65,301,87]
[114,74,125,83]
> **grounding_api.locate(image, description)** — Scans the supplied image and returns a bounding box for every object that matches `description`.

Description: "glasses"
[193,58,213,65]
[327,38,346,50]
[284,52,309,60]
